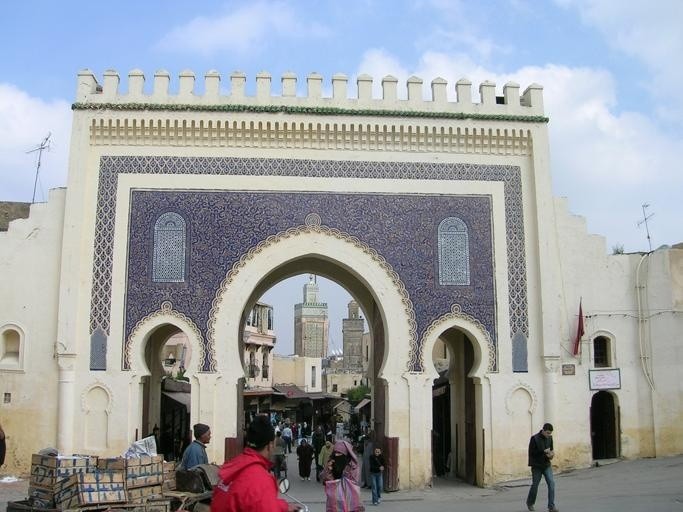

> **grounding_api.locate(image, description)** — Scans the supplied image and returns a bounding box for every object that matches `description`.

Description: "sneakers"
[550,506,559,512]
[301,477,310,480]
[528,504,535,511]
[374,498,380,505]
[285,450,292,454]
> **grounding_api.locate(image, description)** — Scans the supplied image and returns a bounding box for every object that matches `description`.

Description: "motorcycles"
[279,478,308,512]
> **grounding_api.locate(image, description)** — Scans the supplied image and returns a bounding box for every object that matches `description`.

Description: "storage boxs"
[29,453,177,510]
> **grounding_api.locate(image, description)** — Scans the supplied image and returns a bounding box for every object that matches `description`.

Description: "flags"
[573,304,584,355]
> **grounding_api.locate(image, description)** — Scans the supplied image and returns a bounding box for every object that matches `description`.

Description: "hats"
[248,416,274,443]
[194,424,209,438]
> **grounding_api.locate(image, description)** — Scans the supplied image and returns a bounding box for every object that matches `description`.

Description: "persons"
[211,416,302,512]
[275,413,335,485]
[526,423,559,512]
[320,441,366,512]
[180,423,211,469]
[369,448,386,504]
